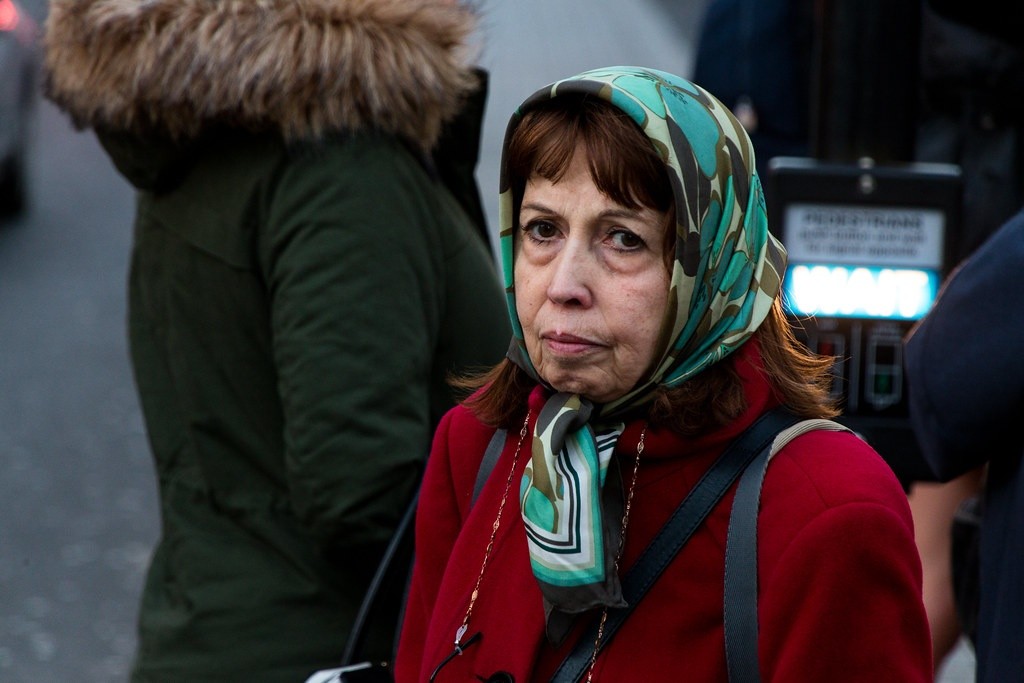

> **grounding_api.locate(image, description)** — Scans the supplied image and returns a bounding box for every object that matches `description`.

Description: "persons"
[43,0,512,682]
[393,68,934,682]
[902,213,1024,682]
